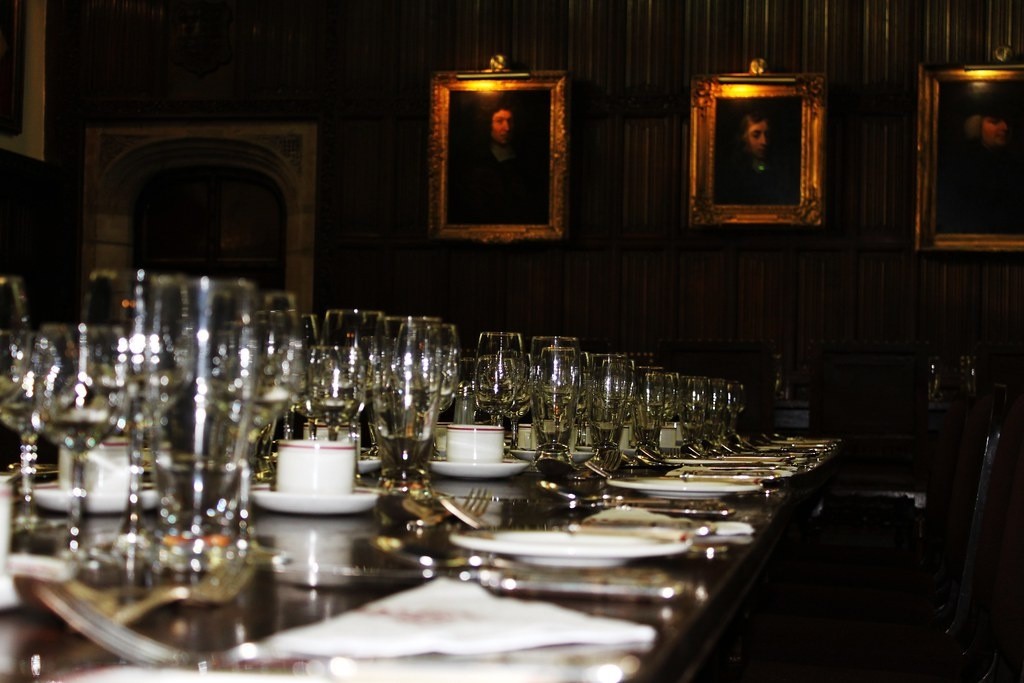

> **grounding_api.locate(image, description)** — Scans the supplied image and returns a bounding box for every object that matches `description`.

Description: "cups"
[0,268,748,604]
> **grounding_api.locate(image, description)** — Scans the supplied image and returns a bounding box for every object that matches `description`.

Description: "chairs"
[714,357,1024,683]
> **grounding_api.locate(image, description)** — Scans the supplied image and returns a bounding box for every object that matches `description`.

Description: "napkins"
[569,504,756,538]
[247,574,659,683]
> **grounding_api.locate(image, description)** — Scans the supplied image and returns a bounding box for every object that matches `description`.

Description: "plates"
[447,433,838,576]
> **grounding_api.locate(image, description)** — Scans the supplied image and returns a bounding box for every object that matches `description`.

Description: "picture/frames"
[689,72,827,226]
[428,53,572,244]
[914,63,1024,251]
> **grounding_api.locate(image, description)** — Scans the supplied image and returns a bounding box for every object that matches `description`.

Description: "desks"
[0,430,858,683]
